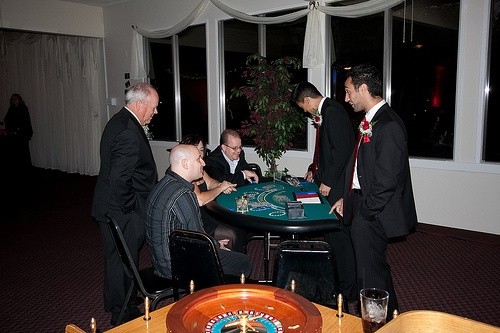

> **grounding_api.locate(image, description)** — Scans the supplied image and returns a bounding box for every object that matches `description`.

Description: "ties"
[348,130,362,197]
[312,126,320,175]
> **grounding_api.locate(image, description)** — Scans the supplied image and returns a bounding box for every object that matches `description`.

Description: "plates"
[166,284,322,333]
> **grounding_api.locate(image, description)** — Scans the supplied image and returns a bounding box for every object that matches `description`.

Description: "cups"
[360,288,389,333]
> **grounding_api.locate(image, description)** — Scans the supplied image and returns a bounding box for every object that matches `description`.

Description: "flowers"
[358,119,373,138]
[311,110,324,128]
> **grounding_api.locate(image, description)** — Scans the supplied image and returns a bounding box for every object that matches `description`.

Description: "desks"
[203,182,342,286]
[102,287,364,333]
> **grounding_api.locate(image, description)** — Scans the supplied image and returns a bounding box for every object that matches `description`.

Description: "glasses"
[224,144,243,150]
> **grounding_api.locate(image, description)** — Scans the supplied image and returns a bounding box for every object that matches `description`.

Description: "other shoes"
[104,296,144,312]
[111,307,144,326]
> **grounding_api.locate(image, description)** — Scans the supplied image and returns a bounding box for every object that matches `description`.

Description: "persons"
[91,82,159,324]
[205,130,259,187]
[144,144,252,280]
[294,82,357,301]
[328,63,418,323]
[4,92,34,170]
[179,136,237,247]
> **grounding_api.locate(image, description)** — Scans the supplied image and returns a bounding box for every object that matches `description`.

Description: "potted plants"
[232,54,306,183]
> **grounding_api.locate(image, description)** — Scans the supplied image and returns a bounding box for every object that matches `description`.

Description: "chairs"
[104,214,184,328]
[274,239,349,314]
[167,229,256,302]
